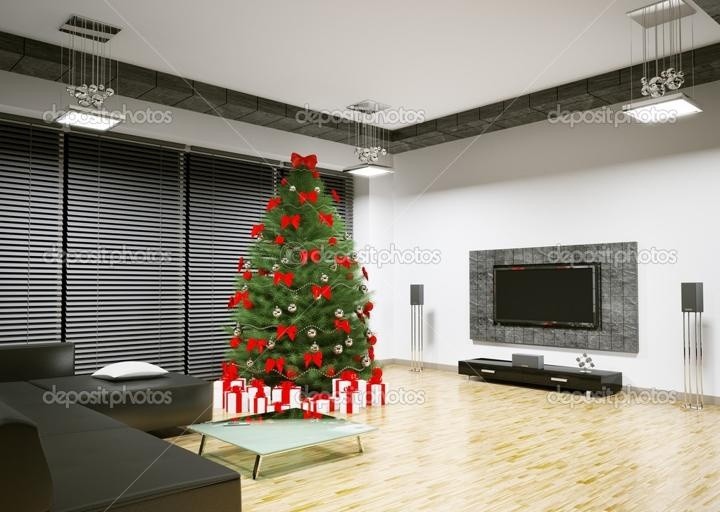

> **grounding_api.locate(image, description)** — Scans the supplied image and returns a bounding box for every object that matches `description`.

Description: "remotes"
[223,422,249,426]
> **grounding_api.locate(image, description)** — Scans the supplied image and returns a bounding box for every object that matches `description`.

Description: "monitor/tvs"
[493,262,602,332]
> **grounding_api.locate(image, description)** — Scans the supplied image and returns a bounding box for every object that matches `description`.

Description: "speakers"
[682,282,703,312]
[512,353,544,370]
[410,284,424,305]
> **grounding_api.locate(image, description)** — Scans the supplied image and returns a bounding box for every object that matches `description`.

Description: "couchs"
[1,337,245,511]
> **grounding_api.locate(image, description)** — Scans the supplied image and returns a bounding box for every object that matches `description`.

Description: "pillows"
[92,360,168,381]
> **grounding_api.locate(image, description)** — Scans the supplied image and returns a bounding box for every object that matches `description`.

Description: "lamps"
[40,15,125,135]
[342,95,397,177]
[623,0,706,127]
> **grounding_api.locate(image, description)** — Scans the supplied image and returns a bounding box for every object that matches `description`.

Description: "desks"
[459,357,623,398]
